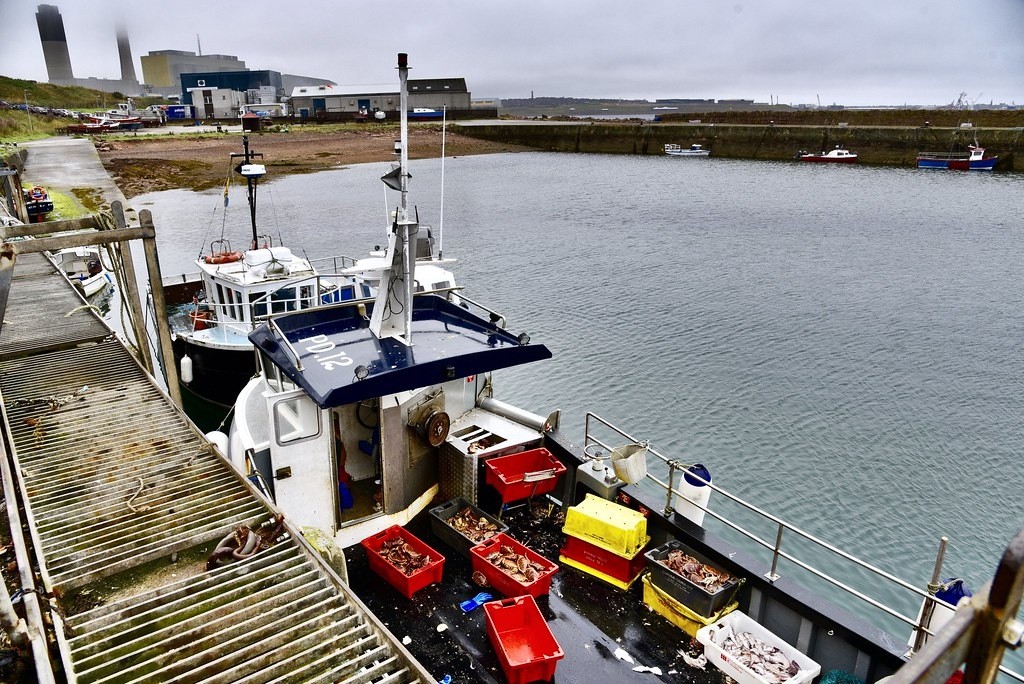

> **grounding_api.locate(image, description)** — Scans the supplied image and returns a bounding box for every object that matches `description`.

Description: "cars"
[11,104,92,119]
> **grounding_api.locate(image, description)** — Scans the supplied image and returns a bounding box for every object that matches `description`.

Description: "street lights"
[23,89,33,132]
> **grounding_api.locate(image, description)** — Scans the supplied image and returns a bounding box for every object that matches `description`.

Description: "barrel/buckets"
[189,311,207,330]
[609,440,649,483]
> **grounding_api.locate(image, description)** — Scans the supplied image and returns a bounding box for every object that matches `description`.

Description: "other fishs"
[719,627,801,684]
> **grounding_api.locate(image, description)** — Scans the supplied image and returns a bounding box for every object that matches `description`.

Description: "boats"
[32,250,112,304]
[203,51,1024,684]
[407,108,443,116]
[66,122,121,131]
[916,144,999,171]
[7,188,54,217]
[144,134,461,412]
[665,143,711,156]
[86,103,169,129]
[801,149,858,163]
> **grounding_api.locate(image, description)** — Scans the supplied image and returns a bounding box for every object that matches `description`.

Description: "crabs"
[379,537,429,576]
[667,548,728,592]
[489,543,547,586]
[446,509,496,541]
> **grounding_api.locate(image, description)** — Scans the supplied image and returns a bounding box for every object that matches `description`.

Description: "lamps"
[518,332,530,347]
[352,364,370,384]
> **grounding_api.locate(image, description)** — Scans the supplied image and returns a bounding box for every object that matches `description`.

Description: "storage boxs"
[470,532,560,600]
[361,524,445,599]
[482,594,564,684]
[429,496,510,562]
[484,446,567,504]
[557,493,744,638]
[695,609,821,684]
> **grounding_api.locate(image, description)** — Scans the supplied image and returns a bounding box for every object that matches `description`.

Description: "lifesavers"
[204,250,243,264]
[29,186,46,201]
